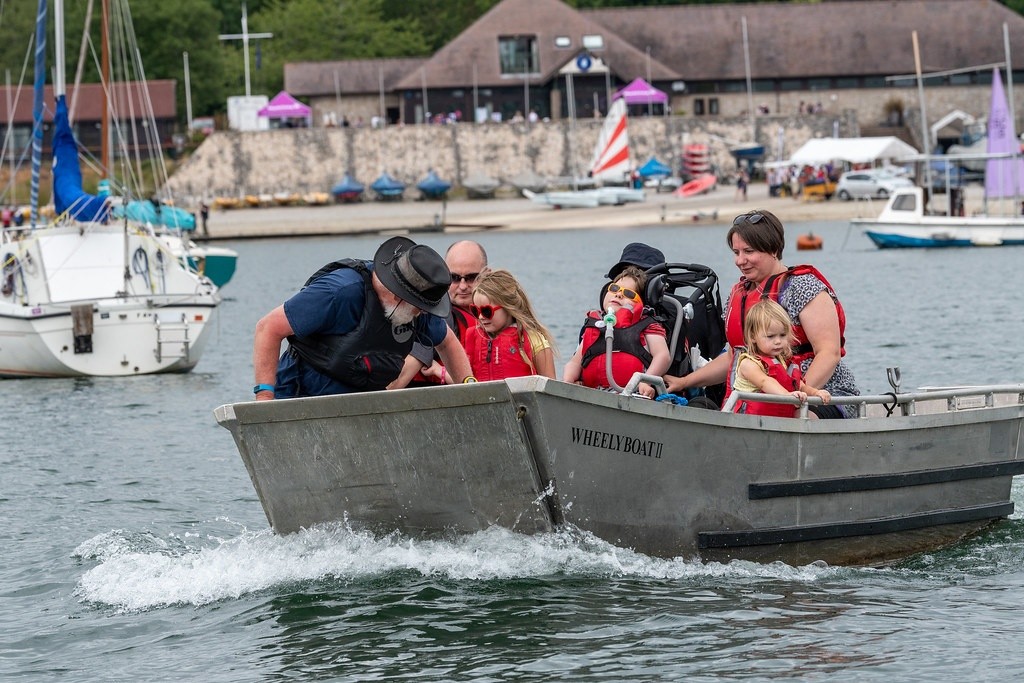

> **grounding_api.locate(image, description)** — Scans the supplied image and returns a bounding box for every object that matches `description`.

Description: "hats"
[373,237,452,318]
[604,243,665,280]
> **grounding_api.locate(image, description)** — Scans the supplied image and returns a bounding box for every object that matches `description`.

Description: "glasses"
[608,283,641,303]
[733,213,768,224]
[469,304,503,320]
[450,272,479,284]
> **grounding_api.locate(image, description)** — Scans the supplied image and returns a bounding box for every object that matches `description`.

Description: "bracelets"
[442,366,447,385]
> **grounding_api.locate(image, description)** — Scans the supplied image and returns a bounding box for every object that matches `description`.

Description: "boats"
[415,170,452,198]
[460,171,501,196]
[673,142,718,196]
[194,188,329,209]
[332,171,365,202]
[370,169,407,197]
[850,21,1024,248]
[212,366,1024,566]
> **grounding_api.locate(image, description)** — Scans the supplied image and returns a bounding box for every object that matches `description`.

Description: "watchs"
[463,377,480,383]
[252,385,277,394]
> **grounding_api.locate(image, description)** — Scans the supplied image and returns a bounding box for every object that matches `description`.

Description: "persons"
[664,211,863,420]
[739,165,858,199]
[3,206,24,238]
[324,110,540,129]
[722,300,832,419]
[253,237,474,401]
[423,269,556,386]
[563,268,671,399]
[760,100,822,115]
[411,240,489,382]
[200,202,209,234]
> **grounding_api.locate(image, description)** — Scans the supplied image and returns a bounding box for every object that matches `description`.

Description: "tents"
[612,78,669,117]
[259,92,313,128]
[792,137,920,176]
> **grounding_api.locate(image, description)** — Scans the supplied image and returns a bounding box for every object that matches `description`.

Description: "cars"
[836,169,916,202]
[192,117,215,136]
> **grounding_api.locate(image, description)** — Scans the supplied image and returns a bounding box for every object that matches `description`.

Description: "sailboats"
[0,0,239,378]
[518,98,649,207]
